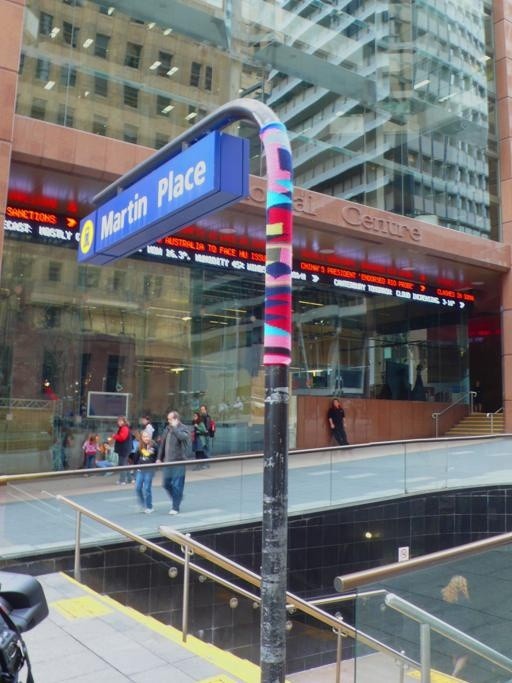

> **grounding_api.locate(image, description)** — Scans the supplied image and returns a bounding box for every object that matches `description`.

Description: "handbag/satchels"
[195,422,209,436]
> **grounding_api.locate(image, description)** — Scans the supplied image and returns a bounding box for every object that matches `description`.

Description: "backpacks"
[204,415,216,438]
[129,431,140,454]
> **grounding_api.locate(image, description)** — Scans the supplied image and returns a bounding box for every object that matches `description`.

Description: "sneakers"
[84,465,210,515]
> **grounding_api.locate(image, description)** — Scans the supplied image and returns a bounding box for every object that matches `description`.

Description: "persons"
[155,409,193,516]
[325,398,352,451]
[48,401,217,487]
[133,429,160,513]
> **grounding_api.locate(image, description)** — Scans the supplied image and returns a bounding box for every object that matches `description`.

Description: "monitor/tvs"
[86,390,133,421]
